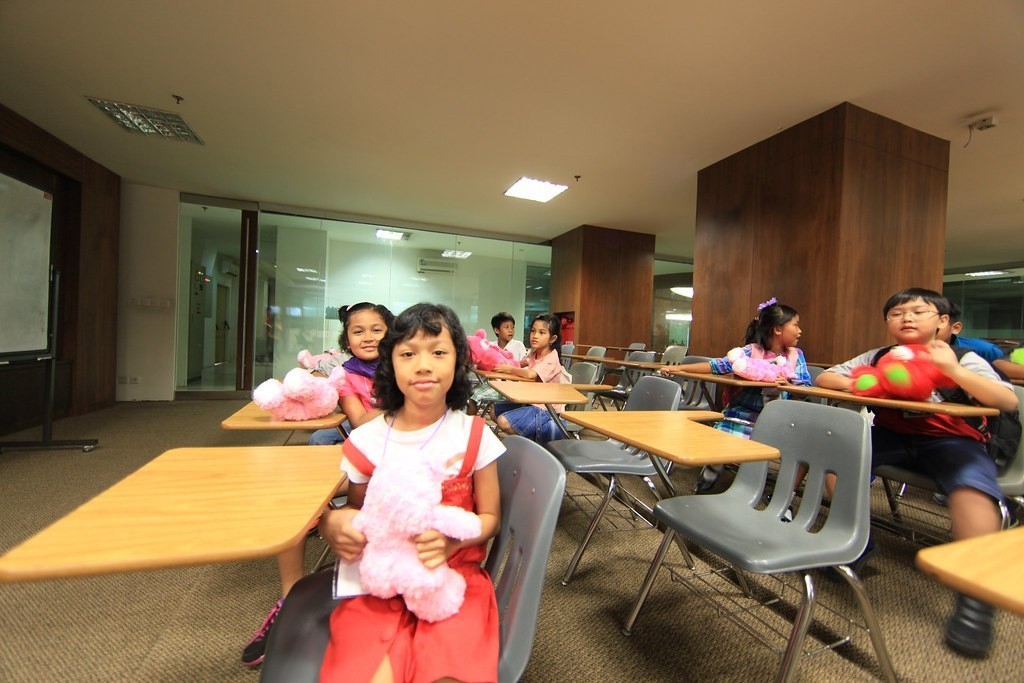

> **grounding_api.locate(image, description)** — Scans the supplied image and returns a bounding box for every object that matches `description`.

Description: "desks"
[668,368,781,389]
[601,358,667,370]
[779,381,1002,419]
[605,344,642,351]
[221,402,346,431]
[488,378,613,404]
[559,352,613,363]
[914,526,1024,611]
[559,405,781,495]
[577,344,594,348]
[0,443,344,599]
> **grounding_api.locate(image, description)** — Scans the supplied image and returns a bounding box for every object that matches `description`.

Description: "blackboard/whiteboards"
[0,171,61,366]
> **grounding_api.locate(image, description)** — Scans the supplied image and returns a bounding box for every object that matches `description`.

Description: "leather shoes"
[694,466,720,495]
[781,506,793,521]
[765,495,772,505]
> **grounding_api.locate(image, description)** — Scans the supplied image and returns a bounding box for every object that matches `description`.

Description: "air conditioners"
[416,257,459,278]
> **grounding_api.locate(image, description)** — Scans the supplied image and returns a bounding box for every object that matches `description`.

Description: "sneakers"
[241,599,283,666]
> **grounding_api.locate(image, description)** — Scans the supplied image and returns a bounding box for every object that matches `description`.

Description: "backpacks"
[872,344,1020,478]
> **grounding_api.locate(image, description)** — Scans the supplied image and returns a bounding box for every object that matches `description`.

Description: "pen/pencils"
[525,349,528,356]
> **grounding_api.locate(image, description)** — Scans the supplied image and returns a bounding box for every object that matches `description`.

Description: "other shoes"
[826,542,880,583]
[946,595,993,657]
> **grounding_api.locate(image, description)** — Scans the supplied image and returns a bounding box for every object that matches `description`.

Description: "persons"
[465,312,572,445]
[240,301,507,682]
[815,287,1024,657]
[658,296,812,522]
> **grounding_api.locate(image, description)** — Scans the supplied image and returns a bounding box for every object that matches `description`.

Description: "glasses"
[885,308,940,321]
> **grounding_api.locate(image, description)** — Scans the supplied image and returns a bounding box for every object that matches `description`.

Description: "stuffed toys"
[297,349,340,368]
[726,346,798,382]
[848,344,958,399]
[1009,348,1024,365]
[352,454,482,626]
[251,366,344,421]
[465,328,519,371]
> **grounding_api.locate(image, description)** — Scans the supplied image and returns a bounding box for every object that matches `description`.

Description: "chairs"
[259,342,1024,683]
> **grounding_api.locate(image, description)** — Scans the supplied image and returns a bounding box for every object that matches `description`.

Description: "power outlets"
[119,376,128,383]
[130,376,140,384]
